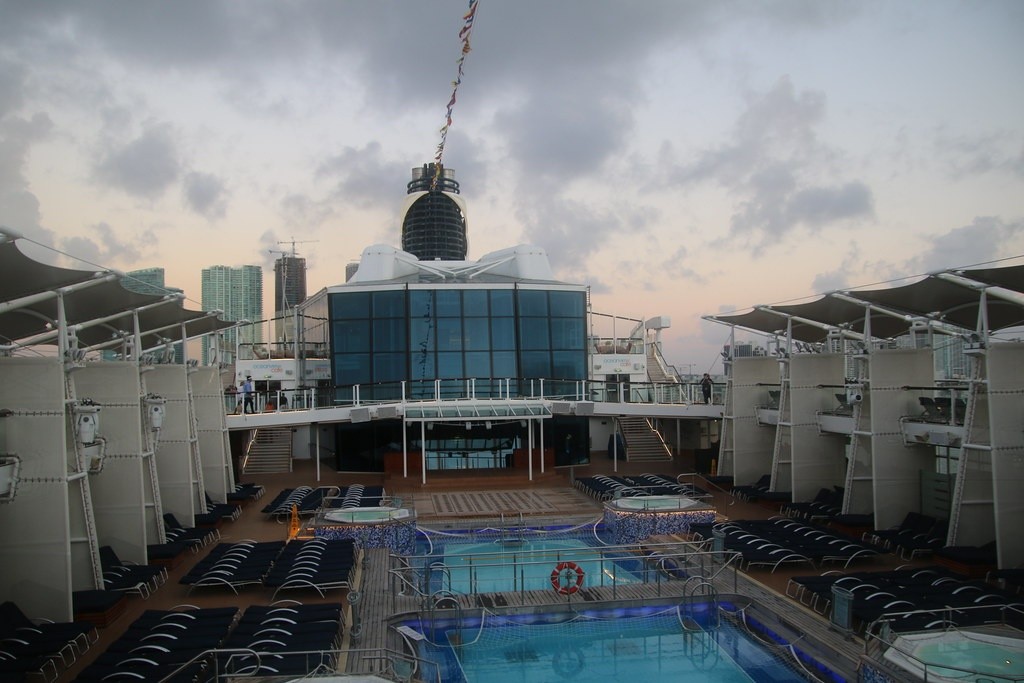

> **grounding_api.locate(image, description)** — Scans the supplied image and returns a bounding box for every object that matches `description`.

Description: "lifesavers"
[551,646,585,679]
[550,562,584,596]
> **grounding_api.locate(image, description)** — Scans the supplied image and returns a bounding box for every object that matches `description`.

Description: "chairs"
[767,391,780,408]
[832,394,853,414]
[574,472,1024,640]
[594,343,633,354]
[908,397,965,426]
[0,483,387,683]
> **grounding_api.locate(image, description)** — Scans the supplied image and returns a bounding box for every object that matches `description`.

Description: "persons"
[242,376,257,413]
[279,393,289,411]
[700,373,713,404]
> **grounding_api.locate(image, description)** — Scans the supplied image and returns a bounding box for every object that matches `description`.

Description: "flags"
[430,0,481,192]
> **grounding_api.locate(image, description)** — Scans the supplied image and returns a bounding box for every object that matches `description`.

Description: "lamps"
[537,418,541,423]
[466,422,471,430]
[485,421,493,429]
[520,420,526,427]
[407,421,412,426]
[427,422,434,430]
[913,432,930,443]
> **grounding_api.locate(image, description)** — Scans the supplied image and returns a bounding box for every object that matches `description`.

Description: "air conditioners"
[349,406,398,424]
[552,401,595,416]
